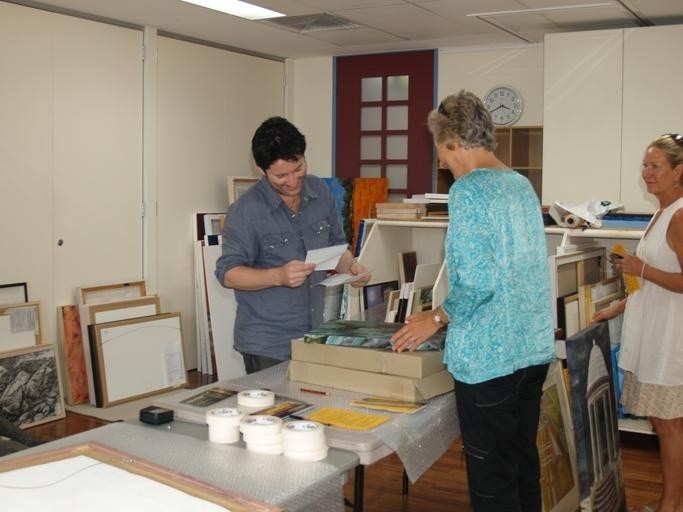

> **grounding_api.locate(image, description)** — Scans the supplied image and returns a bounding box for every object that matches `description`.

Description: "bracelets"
[641,263,648,283]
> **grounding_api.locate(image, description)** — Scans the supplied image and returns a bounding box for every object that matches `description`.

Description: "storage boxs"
[284,359,455,406]
[290,337,446,376]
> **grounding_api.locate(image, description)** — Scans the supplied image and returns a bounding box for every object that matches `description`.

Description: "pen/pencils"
[299,389,326,395]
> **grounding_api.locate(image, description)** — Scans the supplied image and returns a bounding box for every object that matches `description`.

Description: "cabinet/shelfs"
[434,125,540,205]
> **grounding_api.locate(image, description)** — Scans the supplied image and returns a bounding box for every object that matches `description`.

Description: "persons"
[591,132,683,512]
[214,116,372,375]
[389,88,556,512]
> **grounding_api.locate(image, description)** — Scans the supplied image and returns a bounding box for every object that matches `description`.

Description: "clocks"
[481,84,524,129]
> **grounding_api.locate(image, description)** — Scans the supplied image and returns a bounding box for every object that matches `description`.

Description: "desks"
[0,413,359,509]
[339,215,656,323]
[123,359,466,510]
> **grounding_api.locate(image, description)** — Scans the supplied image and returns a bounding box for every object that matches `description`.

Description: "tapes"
[236,390,275,410]
[206,407,330,462]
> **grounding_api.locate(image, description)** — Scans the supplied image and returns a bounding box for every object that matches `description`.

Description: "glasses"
[661,132,683,149]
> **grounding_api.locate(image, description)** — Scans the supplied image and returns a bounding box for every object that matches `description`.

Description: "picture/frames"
[357,248,438,324]
[529,359,582,511]
[0,277,188,437]
[193,175,262,241]
[541,241,624,337]
[609,342,629,420]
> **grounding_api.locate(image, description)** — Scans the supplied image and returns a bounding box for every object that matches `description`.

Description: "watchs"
[434,309,447,326]
